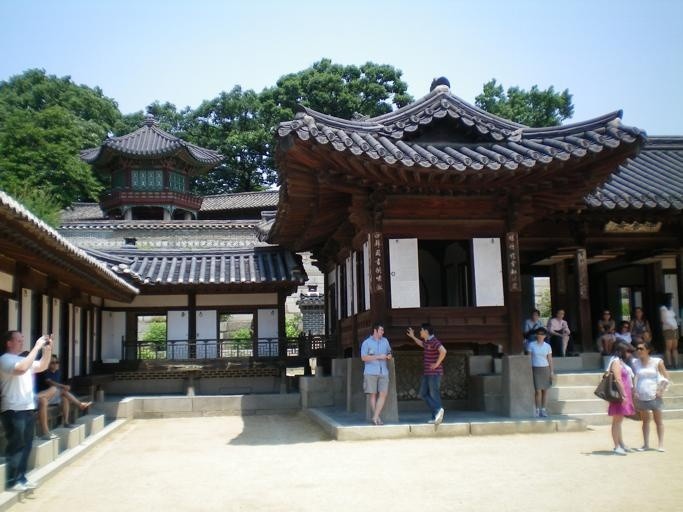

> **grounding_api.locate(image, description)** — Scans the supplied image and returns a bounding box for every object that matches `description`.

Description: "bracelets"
[550,371,554,375]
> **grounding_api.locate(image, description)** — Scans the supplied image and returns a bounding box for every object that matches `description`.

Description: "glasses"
[636,347,644,351]
[50,360,60,365]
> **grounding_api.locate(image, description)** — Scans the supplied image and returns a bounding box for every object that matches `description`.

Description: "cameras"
[45,339,50,345]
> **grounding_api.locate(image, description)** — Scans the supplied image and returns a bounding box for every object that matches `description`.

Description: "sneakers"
[537,408,540,417]
[5,480,38,492]
[63,423,79,429]
[79,401,93,411]
[657,446,666,452]
[666,364,680,369]
[541,409,549,417]
[371,415,384,425]
[613,443,634,455]
[427,407,445,424]
[637,445,650,451]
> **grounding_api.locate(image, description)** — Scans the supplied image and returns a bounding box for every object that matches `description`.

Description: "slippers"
[39,432,61,441]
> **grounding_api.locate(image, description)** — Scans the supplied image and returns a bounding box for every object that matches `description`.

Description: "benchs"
[35,385,96,437]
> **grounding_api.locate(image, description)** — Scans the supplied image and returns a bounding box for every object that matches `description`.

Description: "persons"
[659,292,680,370]
[525,327,554,418]
[546,308,579,357]
[605,339,634,455]
[596,306,652,355]
[43,354,93,429]
[360,322,393,425]
[524,309,550,345]
[407,322,447,425]
[18,351,57,439]
[632,341,669,452]
[0,330,54,492]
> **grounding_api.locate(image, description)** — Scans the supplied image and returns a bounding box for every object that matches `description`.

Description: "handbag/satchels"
[593,359,624,403]
